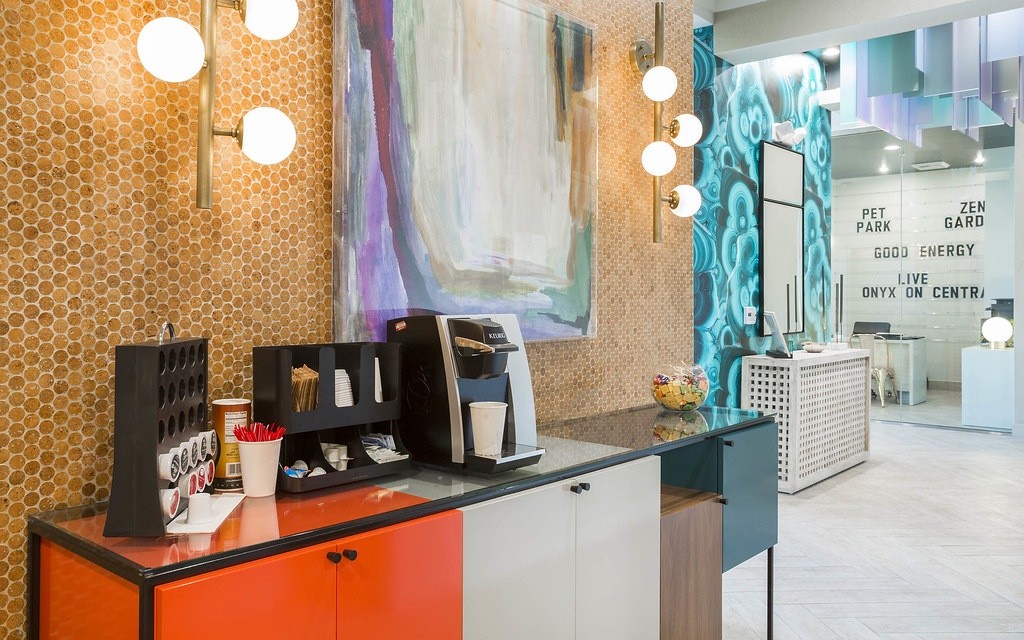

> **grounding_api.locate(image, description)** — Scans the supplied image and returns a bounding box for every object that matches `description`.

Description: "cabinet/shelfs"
[38,509,463,640]
[659,421,778,572]
[660,485,728,640]
[462,455,660,640]
[253,342,412,492]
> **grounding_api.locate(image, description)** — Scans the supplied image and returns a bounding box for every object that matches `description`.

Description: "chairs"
[850,334,899,408]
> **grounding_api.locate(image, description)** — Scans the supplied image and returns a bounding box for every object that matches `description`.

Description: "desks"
[874,337,927,406]
[741,348,872,494]
[962,345,1014,429]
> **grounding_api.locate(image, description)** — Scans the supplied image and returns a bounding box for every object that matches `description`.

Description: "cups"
[469,401,509,456]
[237,437,283,498]
[211,398,251,492]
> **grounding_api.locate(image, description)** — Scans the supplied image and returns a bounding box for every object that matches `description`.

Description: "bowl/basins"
[653,411,709,448]
[650,363,709,413]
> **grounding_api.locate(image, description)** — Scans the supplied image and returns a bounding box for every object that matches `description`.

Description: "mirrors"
[760,141,805,338]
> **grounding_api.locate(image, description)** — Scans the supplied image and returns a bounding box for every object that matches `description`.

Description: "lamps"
[982,316,1013,349]
[630,1,701,242]
[138,1,296,209]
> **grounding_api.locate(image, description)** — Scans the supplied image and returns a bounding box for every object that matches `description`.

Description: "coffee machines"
[386,314,548,476]
